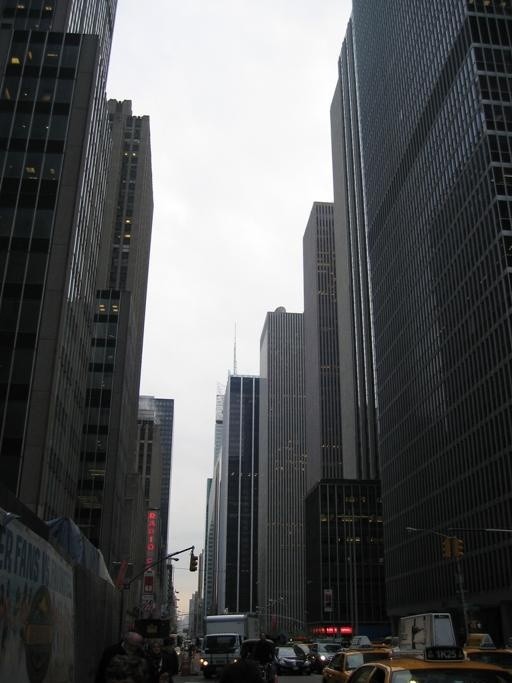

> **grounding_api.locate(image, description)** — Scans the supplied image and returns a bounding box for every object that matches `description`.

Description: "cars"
[345,613,512,683]
[178,637,203,676]
[322,635,393,682]
[461,634,512,669]
[273,641,344,676]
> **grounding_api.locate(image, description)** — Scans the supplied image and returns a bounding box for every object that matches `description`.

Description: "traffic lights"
[190,553,198,571]
[455,538,465,558]
[440,535,451,559]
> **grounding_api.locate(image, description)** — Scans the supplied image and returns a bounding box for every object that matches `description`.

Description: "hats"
[126,631,144,647]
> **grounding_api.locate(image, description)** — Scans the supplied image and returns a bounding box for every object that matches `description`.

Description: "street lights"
[404,525,471,644]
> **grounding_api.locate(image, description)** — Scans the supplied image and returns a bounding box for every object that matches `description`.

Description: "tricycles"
[228,638,278,682]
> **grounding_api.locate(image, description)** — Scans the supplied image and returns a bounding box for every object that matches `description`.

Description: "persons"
[216,644,272,683]
[257,631,268,642]
[174,643,180,665]
[93,628,158,682]
[161,638,179,676]
[148,641,173,682]
[104,653,151,682]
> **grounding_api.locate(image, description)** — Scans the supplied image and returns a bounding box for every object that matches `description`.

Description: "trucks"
[195,612,262,679]
